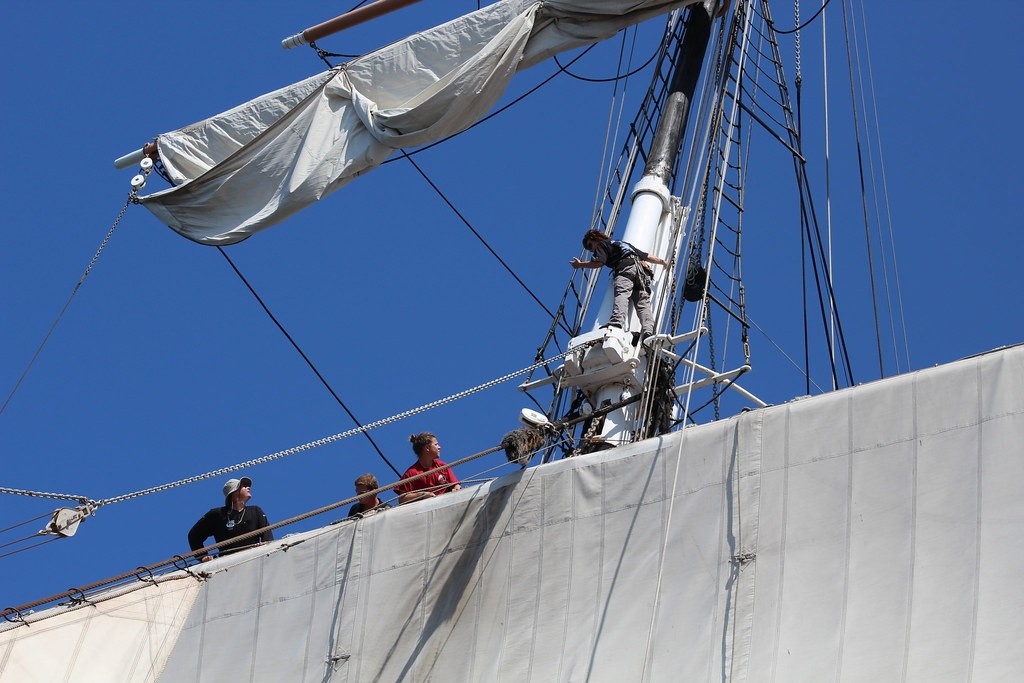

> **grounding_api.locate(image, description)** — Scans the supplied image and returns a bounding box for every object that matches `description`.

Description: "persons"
[347,474,392,518]
[188,477,274,562]
[393,432,461,503]
[568,229,667,341]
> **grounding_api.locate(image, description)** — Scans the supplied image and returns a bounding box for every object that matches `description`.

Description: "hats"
[223,476,252,507]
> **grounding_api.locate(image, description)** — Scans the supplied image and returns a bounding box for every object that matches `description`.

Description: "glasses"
[587,240,593,250]
[355,488,371,495]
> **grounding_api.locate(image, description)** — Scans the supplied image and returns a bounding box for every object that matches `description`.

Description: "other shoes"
[631,331,650,347]
[599,322,622,329]
